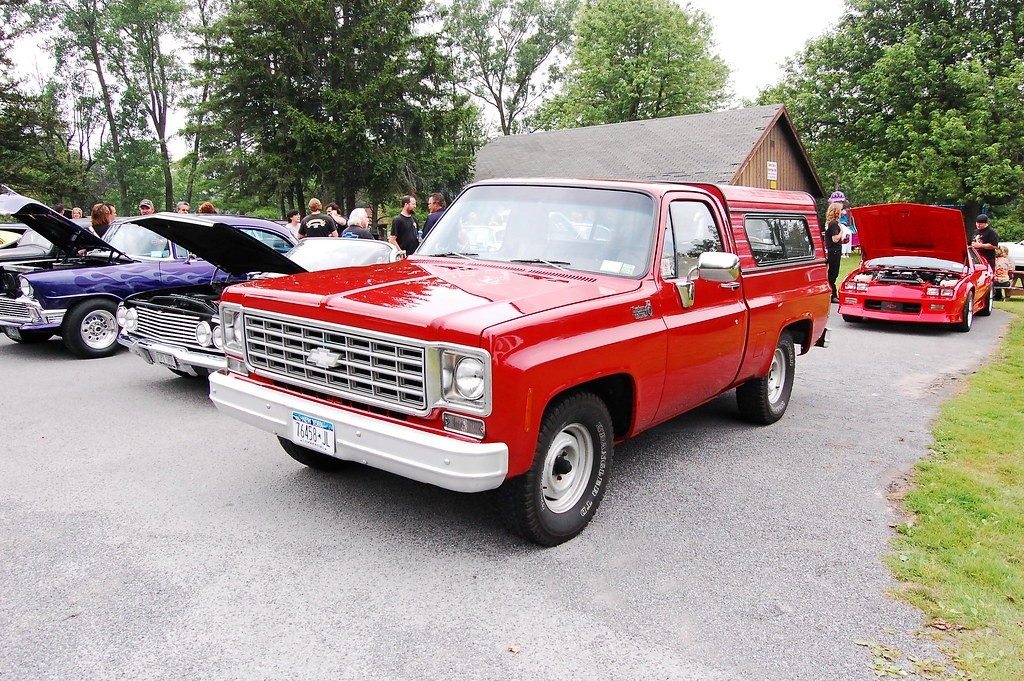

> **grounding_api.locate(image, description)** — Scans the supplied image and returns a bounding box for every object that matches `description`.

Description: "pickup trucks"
[209,176,832,549]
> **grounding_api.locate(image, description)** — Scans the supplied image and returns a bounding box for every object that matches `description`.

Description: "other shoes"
[830,297,840,304]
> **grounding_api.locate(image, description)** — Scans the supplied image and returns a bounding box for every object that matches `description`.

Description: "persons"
[54,203,64,215]
[838,209,852,258]
[993,245,1015,299]
[421,194,459,248]
[297,198,346,240]
[825,203,849,303]
[168,201,189,258]
[971,214,998,274]
[77,203,112,256]
[389,195,419,255]
[128,199,167,253]
[198,202,215,215]
[105,203,117,221]
[340,208,375,239]
[285,210,301,239]
[72,207,83,219]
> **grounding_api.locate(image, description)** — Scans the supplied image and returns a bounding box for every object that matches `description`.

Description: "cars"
[0,183,300,360]
[115,212,405,380]
[838,204,994,333]
[0,218,130,269]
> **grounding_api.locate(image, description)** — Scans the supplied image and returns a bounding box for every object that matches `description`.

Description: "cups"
[846,234,850,242]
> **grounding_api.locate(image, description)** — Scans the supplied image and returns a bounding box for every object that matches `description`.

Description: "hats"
[976,214,989,223]
[139,198,152,207]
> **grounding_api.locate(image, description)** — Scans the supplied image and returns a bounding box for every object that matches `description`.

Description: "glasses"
[140,207,149,210]
[180,209,189,212]
